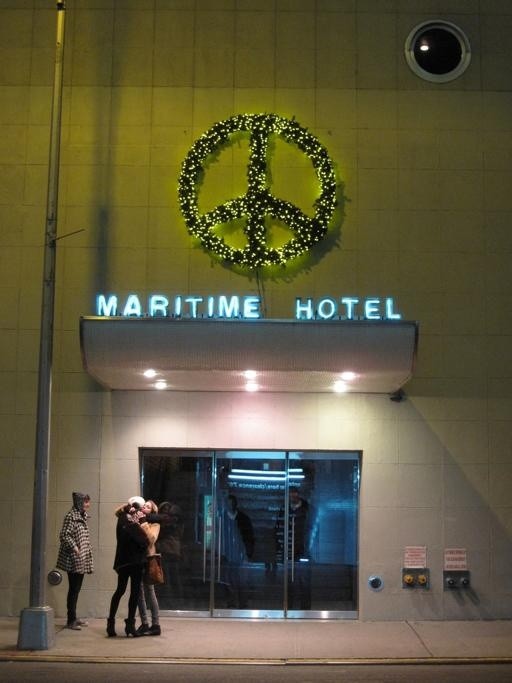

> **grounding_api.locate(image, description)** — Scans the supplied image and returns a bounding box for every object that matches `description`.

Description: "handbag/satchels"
[143,554,164,585]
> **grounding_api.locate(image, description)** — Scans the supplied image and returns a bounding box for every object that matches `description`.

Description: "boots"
[124,618,139,636]
[106,617,117,637]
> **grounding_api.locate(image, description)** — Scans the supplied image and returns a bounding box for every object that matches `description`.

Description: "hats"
[128,496,146,508]
[72,491,87,508]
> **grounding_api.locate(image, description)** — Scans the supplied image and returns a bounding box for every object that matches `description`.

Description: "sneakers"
[69,618,88,629]
[136,624,160,635]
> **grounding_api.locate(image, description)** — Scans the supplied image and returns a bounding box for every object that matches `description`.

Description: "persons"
[274,484,318,608]
[159,500,178,608]
[219,493,257,607]
[106,495,172,638]
[164,501,188,608]
[54,489,95,631]
[135,495,165,637]
